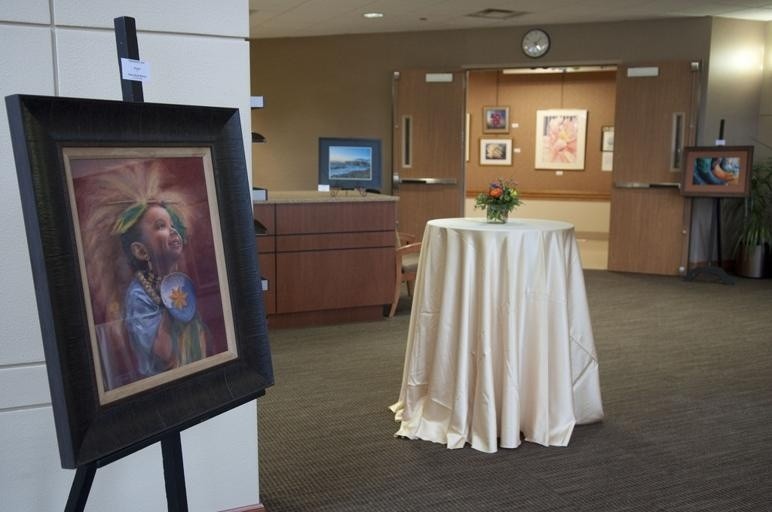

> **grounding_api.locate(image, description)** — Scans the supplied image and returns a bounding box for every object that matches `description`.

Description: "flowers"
[474,175,524,219]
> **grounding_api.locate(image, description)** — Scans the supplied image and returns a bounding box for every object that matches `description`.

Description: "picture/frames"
[480,138,512,166]
[679,145,754,198]
[600,125,615,152]
[319,137,381,189]
[482,105,511,134]
[4,93,275,469]
[534,108,588,171]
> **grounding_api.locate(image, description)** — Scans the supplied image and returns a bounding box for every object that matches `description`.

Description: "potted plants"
[722,161,772,278]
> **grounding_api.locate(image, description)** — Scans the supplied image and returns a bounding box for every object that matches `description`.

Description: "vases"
[487,205,509,224]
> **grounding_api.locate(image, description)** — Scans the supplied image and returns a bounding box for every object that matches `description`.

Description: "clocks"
[520,28,551,58]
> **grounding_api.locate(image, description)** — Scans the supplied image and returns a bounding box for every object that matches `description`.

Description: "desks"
[248,196,400,329]
[387,218,605,454]
